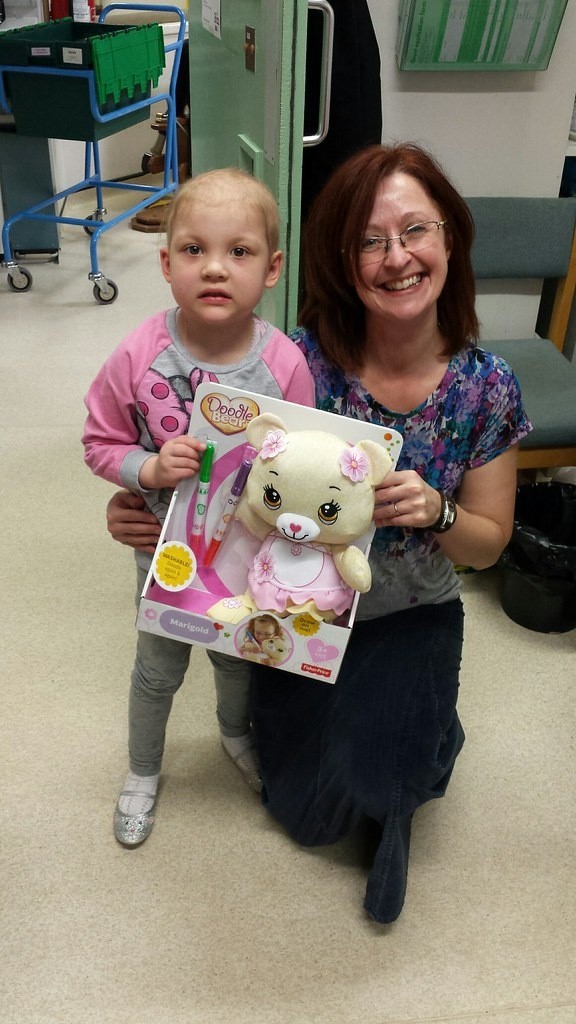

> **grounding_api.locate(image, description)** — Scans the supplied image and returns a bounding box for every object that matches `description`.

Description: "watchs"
[414,490,457,533]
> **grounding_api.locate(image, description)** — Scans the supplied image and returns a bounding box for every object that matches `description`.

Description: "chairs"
[445,196,576,470]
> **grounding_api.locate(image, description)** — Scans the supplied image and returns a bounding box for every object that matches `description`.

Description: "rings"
[394,502,400,516]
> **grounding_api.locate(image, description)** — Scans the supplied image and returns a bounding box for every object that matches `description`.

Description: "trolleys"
[0,3,188,307]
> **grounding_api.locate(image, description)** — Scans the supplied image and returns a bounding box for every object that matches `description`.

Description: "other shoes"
[221,740,265,793]
[113,782,157,846]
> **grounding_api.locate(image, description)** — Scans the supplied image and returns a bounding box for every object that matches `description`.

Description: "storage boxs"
[0,20,167,142]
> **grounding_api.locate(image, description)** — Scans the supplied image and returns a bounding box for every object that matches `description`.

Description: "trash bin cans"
[499,482,576,633]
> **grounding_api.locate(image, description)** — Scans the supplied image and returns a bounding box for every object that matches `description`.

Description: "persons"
[237,614,282,665]
[106,142,533,922]
[82,167,317,846]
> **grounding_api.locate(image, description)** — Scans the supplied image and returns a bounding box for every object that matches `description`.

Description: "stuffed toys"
[206,414,392,623]
[242,636,292,665]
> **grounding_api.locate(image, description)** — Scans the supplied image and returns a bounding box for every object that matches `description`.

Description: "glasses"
[341,220,448,265]
